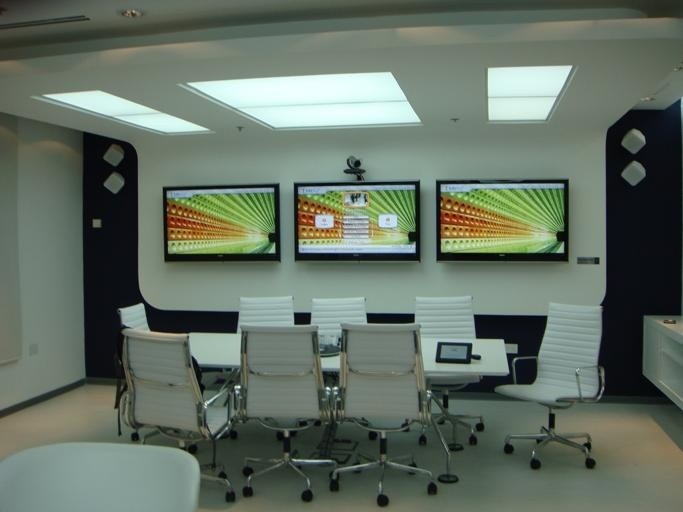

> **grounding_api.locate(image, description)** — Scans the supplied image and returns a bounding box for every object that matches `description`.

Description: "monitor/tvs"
[294,178,421,262]
[440,178,569,261]
[163,182,281,263]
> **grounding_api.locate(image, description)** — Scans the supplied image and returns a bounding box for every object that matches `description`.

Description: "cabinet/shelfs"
[642,315,683,411]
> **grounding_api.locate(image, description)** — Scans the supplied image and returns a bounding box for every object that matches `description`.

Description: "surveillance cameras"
[349,156,360,167]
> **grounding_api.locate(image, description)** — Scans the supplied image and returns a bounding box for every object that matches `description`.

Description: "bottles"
[318,334,336,353]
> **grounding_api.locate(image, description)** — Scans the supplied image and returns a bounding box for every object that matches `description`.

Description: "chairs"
[113,301,155,438]
[401,294,485,450]
[494,301,605,471]
[329,321,439,506]
[308,296,371,340]
[121,328,236,504]
[235,295,297,333]
[0,443,202,512]
[239,323,337,504]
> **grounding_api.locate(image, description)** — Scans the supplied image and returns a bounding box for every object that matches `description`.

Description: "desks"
[185,330,512,467]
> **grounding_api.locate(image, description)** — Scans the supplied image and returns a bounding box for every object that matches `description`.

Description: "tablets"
[436,341,472,363]
